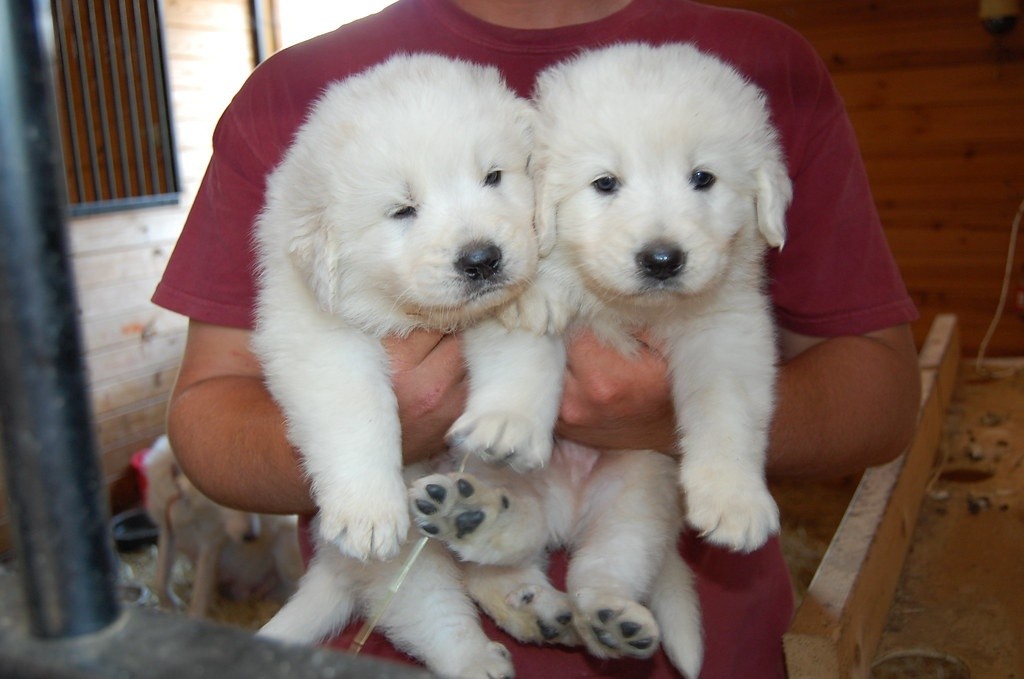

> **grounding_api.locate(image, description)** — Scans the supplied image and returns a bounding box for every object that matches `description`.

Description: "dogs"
[140,432,305,624]
[244,39,793,679]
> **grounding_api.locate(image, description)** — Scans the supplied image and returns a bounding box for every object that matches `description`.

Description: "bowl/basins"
[109,507,159,552]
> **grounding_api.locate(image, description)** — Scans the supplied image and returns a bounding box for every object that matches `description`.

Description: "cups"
[870,649,971,679]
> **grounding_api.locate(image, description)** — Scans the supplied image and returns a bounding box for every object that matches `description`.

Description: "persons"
[148,1,924,678]
